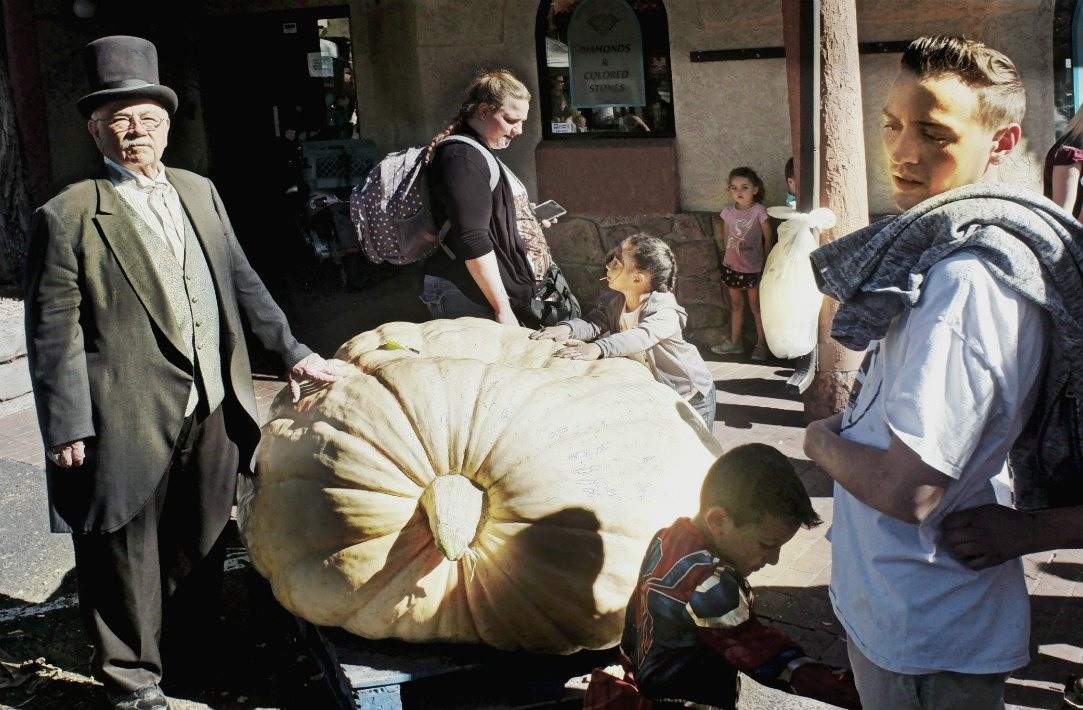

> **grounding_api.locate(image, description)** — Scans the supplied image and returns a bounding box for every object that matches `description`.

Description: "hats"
[74,35,178,118]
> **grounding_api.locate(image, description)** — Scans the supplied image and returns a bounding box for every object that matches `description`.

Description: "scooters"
[280,122,363,294]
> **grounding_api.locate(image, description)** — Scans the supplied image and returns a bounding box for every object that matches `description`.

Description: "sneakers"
[751,344,770,363]
[104,674,170,710]
[711,339,744,355]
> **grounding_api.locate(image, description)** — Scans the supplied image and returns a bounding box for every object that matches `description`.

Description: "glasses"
[95,113,167,131]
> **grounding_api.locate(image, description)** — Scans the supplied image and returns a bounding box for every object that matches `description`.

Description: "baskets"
[302,139,380,188]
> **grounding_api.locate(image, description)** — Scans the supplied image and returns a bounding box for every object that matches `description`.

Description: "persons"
[803,32,1083,710]
[24,35,350,710]
[1043,103,1083,224]
[529,233,716,435]
[550,54,672,133]
[785,157,796,209]
[710,167,773,362]
[408,69,582,330]
[581,443,863,710]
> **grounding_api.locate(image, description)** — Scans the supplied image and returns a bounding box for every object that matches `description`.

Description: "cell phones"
[533,199,567,225]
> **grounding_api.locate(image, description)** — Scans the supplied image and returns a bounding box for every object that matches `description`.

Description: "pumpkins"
[237,319,724,653]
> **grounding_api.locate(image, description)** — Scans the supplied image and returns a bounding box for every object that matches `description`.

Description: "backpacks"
[350,135,500,265]
